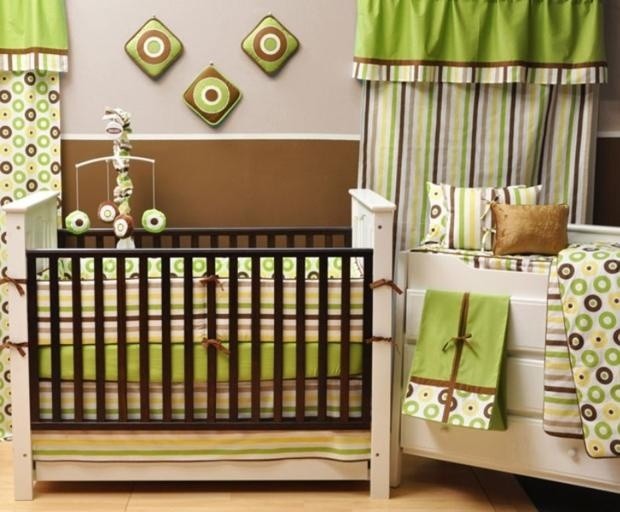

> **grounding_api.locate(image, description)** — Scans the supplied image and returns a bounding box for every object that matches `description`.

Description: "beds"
[3,188,402,498]
[389,222,620,495]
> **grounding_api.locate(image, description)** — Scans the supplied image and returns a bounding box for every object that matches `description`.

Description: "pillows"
[420,182,569,256]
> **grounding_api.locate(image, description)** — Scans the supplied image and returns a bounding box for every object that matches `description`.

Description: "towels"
[401,288,511,430]
